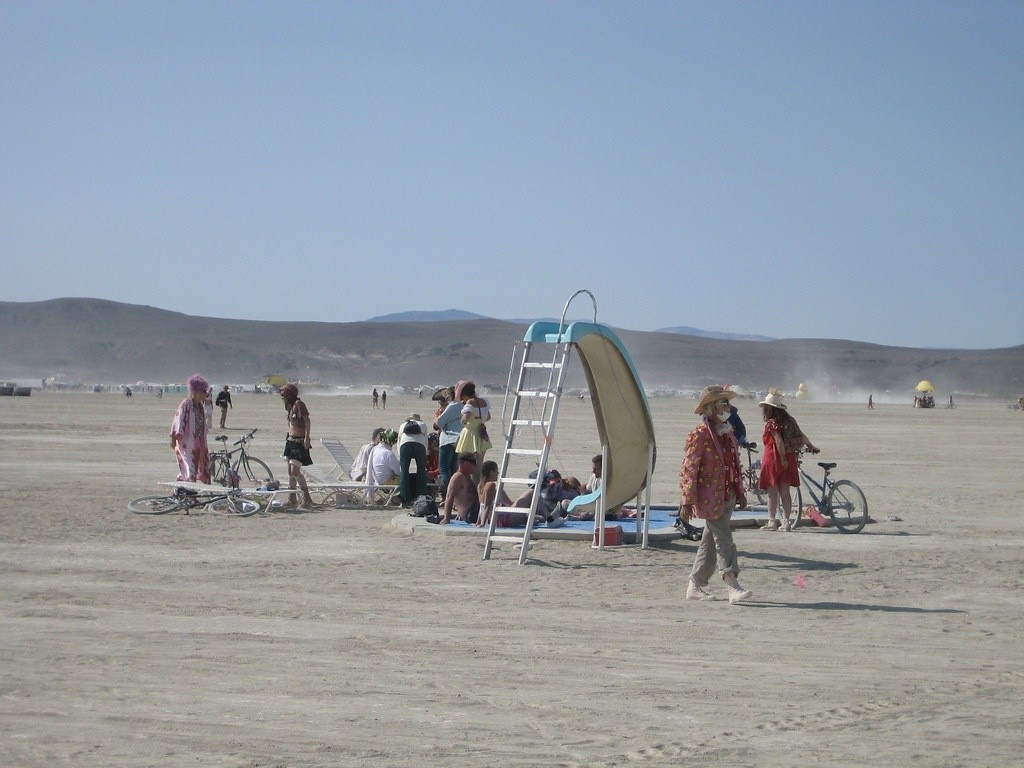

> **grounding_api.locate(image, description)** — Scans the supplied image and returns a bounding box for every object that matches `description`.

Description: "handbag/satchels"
[413,495,438,517]
[479,423,490,441]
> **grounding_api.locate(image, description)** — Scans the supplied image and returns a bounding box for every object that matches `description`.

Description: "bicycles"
[776,448,869,535]
[740,442,769,505]
[208,428,274,487]
[128,458,260,518]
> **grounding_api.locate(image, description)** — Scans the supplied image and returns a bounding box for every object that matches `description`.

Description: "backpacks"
[402,420,425,436]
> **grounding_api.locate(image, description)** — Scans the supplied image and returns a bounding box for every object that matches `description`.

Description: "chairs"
[157,434,445,512]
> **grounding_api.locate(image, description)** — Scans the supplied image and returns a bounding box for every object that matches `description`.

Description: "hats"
[405,413,422,422]
[379,427,398,444]
[454,379,474,402]
[694,385,737,416]
[432,385,449,401]
[758,393,786,409]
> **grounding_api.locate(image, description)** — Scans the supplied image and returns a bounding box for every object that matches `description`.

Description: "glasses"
[549,478,560,485]
[716,400,729,406]
[460,458,478,465]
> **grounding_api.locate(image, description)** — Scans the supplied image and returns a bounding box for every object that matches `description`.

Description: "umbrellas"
[915,381,934,395]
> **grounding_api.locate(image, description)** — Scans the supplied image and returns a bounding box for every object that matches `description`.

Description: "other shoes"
[760,519,791,532]
[282,501,313,510]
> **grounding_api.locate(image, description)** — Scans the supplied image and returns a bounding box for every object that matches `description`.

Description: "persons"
[216,385,233,429]
[868,395,874,410]
[127,388,130,396]
[469,461,568,528]
[948,396,953,408]
[373,389,379,409]
[913,395,935,408]
[679,387,752,604]
[382,391,386,409]
[544,455,644,521]
[279,384,314,510]
[758,393,817,532]
[351,379,491,508]
[439,450,513,525]
[728,405,746,510]
[420,391,423,396]
[170,375,209,504]
[202,386,213,429]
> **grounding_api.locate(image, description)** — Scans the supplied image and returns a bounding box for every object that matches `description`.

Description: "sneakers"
[728,584,752,605]
[551,501,562,518]
[686,581,715,601]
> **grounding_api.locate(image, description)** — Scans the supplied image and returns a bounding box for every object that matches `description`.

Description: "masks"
[716,411,731,422]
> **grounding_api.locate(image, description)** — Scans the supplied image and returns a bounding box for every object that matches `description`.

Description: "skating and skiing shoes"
[547,518,567,528]
